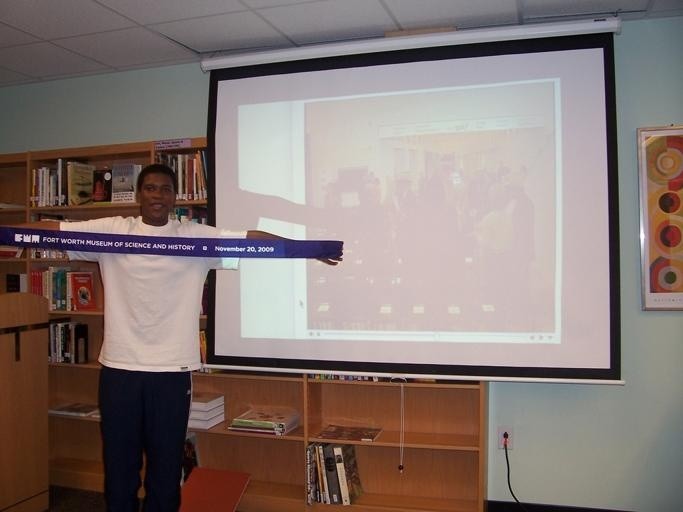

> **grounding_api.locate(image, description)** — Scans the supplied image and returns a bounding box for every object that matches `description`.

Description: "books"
[154,152,209,226]
[30,213,85,259]
[179,432,200,484]
[50,402,101,418]
[187,393,224,432]
[307,373,373,381]
[197,331,222,374]
[30,158,142,208]
[180,468,251,512]
[227,408,299,437]
[306,442,363,505]
[314,425,382,442]
[48,317,89,364]
[0,246,93,312]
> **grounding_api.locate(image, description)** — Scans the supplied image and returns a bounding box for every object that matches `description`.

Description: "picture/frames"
[637,124,683,310]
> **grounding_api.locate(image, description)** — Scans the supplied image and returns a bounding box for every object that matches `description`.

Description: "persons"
[0,164,344,512]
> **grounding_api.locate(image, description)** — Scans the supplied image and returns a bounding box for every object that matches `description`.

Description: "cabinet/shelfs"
[0,292,50,512]
[0,136,488,512]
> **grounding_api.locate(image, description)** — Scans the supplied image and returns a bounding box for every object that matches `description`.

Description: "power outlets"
[498,426,513,450]
